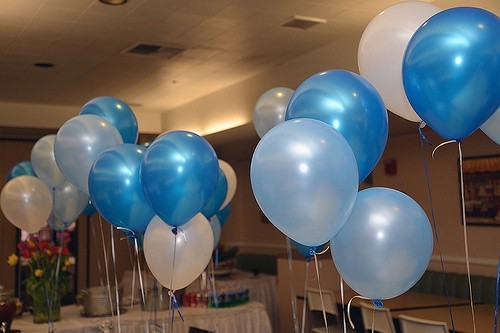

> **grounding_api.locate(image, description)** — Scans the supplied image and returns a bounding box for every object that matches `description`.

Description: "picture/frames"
[458,154,500,226]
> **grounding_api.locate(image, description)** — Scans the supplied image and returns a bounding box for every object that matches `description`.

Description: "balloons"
[0,96,237,293]
[250,69,389,260]
[357,1,500,144]
[330,187,434,308]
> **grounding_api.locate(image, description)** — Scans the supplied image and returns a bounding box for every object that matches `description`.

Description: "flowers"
[7,226,75,316]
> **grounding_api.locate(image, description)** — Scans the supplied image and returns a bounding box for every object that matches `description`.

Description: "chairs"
[398,314,449,333]
[359,302,396,333]
[307,287,357,333]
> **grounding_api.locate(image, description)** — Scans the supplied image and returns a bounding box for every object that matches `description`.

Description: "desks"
[10,300,272,333]
[393,303,500,333]
[297,289,484,310]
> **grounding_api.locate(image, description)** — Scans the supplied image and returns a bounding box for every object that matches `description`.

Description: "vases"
[32,286,60,324]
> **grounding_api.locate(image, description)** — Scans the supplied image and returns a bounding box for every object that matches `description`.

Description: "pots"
[80,283,121,317]
